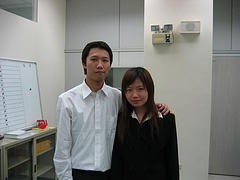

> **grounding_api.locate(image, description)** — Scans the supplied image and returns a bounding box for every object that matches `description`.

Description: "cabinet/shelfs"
[0,127,57,180]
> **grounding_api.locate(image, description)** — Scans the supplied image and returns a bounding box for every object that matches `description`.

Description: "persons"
[52,41,170,180]
[109,67,180,180]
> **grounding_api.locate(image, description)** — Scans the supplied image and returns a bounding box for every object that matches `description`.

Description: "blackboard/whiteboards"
[0,58,44,138]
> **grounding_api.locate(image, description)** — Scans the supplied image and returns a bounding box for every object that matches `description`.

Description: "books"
[4,130,35,140]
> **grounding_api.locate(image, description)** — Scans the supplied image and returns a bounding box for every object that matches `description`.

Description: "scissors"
[22,126,39,131]
